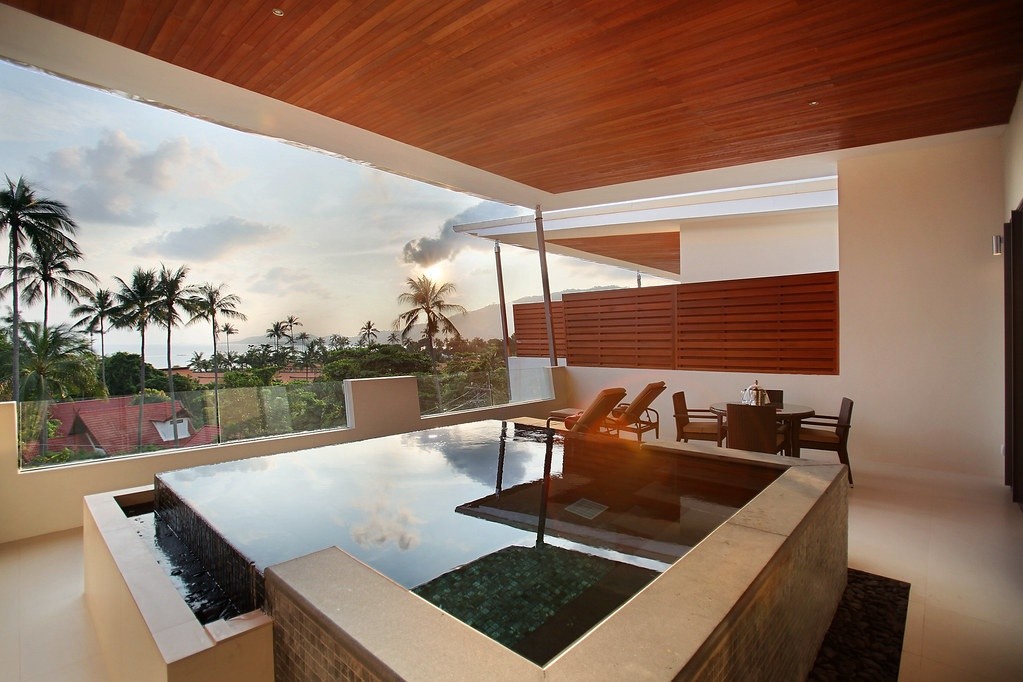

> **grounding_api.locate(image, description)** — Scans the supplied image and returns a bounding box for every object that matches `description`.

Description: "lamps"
[992,236,1005,256]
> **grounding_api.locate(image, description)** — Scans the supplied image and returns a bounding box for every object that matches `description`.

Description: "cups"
[740,389,752,405]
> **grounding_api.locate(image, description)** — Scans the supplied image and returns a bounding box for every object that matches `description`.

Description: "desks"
[712,401,815,458]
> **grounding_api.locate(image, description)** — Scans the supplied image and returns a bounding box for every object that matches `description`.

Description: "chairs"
[672,391,727,447]
[550,380,667,442]
[800,397,854,488]
[745,389,783,456]
[726,404,791,457]
[508,378,626,438]
